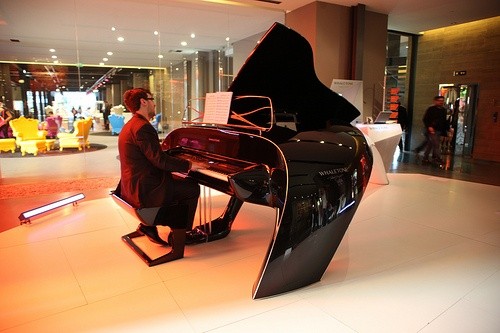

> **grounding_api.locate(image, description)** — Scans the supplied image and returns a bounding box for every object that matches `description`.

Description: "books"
[203,92,233,125]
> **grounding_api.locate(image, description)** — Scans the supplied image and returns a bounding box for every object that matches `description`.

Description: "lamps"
[18,193,85,225]
[87,64,120,94]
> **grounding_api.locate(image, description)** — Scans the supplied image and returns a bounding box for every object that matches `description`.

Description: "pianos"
[158,22,373,303]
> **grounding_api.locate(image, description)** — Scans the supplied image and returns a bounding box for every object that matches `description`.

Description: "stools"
[111,191,188,266]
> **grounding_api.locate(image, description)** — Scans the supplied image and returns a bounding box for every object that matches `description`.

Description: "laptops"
[373,112,393,124]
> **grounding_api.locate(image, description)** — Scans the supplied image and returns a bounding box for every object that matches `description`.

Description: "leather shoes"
[168,230,202,246]
[136,223,168,246]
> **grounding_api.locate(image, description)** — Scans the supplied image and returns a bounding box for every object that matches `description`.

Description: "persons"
[37,106,62,139]
[0,97,13,137]
[118,88,199,246]
[398,97,450,170]
[313,205,320,232]
[72,106,83,119]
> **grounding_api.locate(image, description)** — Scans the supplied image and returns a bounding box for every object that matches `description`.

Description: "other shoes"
[424,159,430,165]
[432,162,439,168]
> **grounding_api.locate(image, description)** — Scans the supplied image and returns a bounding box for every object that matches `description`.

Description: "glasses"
[145,98,155,102]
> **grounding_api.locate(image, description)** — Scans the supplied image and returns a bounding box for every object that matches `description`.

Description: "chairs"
[0,115,95,157]
[151,112,162,131]
[109,104,128,135]
